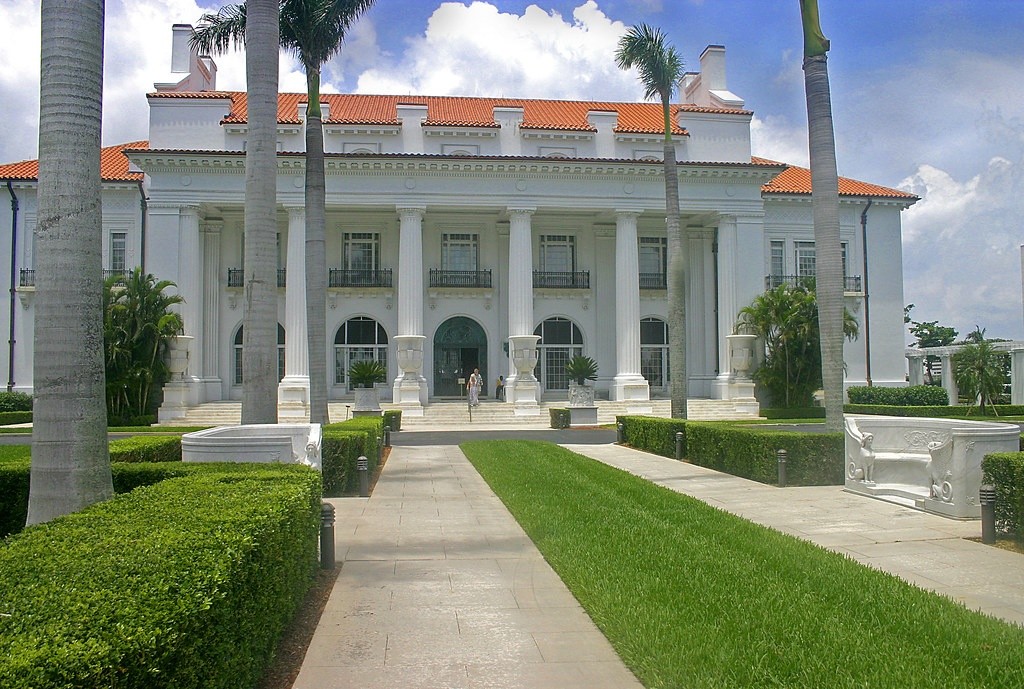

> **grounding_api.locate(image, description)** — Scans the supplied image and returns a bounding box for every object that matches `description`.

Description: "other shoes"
[471,405,473,406]
[476,404,478,406]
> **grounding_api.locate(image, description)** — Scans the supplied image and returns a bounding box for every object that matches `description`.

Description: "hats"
[474,368,479,372]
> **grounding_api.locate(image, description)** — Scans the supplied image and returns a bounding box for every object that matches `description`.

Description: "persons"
[466,368,483,406]
[496,376,504,399]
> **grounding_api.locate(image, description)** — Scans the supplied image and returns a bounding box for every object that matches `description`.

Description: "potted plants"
[564,354,599,406]
[345,359,387,409]
